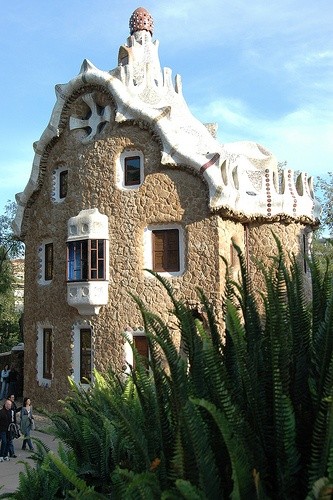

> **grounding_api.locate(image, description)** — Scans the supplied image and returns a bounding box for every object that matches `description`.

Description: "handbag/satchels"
[31,419,35,429]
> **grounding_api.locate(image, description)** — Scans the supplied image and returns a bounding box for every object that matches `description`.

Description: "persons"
[20,397,35,452]
[0,364,24,462]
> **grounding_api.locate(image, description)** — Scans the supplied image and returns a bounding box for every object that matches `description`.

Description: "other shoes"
[0,456,4,462]
[31,448,34,450]
[24,448,28,450]
[4,456,10,461]
[9,453,17,458]
